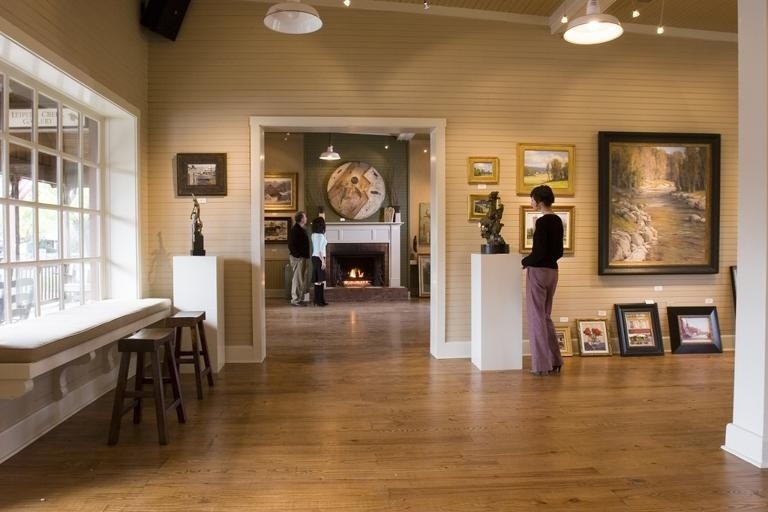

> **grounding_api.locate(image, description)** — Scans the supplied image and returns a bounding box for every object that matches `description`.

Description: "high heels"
[530,365,561,376]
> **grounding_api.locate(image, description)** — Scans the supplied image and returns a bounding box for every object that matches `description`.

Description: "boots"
[313,284,329,306]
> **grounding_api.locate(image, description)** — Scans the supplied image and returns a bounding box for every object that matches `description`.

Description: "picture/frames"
[417,253,430,297]
[264,171,299,211]
[597,130,720,273]
[519,205,575,257]
[264,217,291,244]
[516,142,576,197]
[552,267,737,357]
[468,194,500,221]
[467,157,500,184]
[319,133,341,160]
[177,152,228,197]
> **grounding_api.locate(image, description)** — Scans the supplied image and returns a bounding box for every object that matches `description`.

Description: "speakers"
[141,0,190,43]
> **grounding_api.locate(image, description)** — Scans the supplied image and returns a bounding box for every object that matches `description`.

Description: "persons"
[311,217,329,306]
[521,185,564,376]
[288,211,309,306]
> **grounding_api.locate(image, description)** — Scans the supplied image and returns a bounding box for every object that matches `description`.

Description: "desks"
[0,299,172,402]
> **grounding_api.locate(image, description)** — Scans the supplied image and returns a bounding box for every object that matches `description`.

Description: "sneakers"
[293,301,307,306]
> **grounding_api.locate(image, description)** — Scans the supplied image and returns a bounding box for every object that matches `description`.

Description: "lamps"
[263,0,323,34]
[560,0,624,45]
[424,0,429,9]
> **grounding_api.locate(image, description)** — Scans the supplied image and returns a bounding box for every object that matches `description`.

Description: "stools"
[161,311,214,399]
[107,328,185,446]
